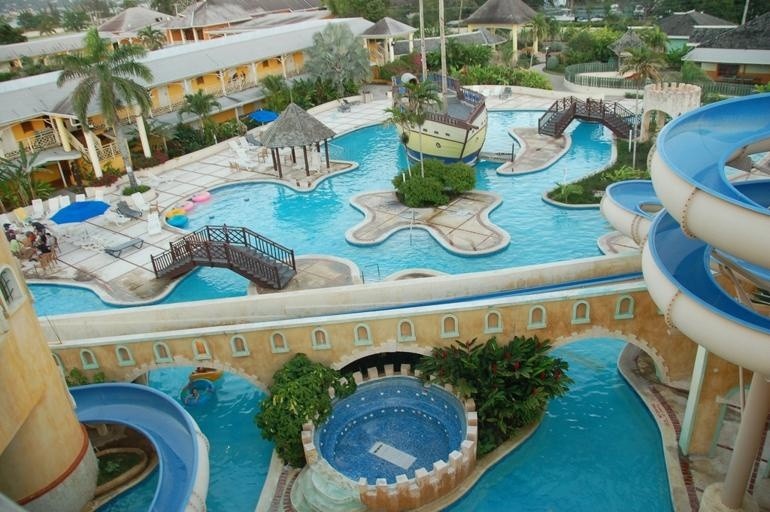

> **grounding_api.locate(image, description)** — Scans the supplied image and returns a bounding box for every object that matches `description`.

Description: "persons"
[181,367,216,405]
[2,220,54,254]
[233,70,246,86]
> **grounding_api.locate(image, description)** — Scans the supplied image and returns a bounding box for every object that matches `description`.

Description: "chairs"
[227,135,322,178]
[472,85,514,103]
[0,191,162,276]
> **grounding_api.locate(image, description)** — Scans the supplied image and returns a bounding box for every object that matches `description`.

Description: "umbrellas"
[51,201,110,241]
[247,107,279,127]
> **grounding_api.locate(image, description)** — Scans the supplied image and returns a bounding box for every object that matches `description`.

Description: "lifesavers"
[182,379,215,408]
[189,367,221,378]
[167,188,213,226]
